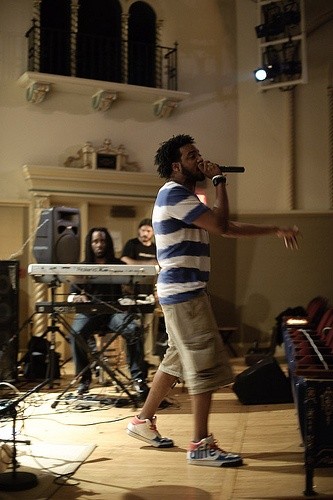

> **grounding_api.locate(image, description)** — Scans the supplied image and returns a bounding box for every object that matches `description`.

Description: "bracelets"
[212,174,223,180]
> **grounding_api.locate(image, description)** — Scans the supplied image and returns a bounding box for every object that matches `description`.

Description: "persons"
[127,135,303,467]
[67,220,160,396]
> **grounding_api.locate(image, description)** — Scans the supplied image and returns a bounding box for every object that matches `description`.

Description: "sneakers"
[188,433,242,467]
[127,415,173,448]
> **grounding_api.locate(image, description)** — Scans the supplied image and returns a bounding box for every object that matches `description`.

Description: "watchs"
[212,176,227,186]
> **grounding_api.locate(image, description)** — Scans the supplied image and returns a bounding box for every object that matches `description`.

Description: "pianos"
[27,264,161,408]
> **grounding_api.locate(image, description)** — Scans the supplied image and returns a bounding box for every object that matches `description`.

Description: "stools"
[87,328,132,391]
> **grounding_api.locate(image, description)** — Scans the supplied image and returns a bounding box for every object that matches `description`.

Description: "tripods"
[13,283,73,389]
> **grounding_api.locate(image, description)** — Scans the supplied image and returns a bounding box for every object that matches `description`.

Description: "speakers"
[32,206,81,263]
[0,259,20,385]
[233,357,296,406]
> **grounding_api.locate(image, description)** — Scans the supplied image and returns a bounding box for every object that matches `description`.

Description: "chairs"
[279,296,333,497]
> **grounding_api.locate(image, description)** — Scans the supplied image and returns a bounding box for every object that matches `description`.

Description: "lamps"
[253,63,278,83]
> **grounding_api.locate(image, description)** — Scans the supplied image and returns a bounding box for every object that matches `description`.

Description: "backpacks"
[16,337,61,381]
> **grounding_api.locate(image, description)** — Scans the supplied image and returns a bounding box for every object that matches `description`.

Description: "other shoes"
[77,383,89,393]
[132,382,149,392]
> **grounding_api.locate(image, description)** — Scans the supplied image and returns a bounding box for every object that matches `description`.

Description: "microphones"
[219,165,245,173]
